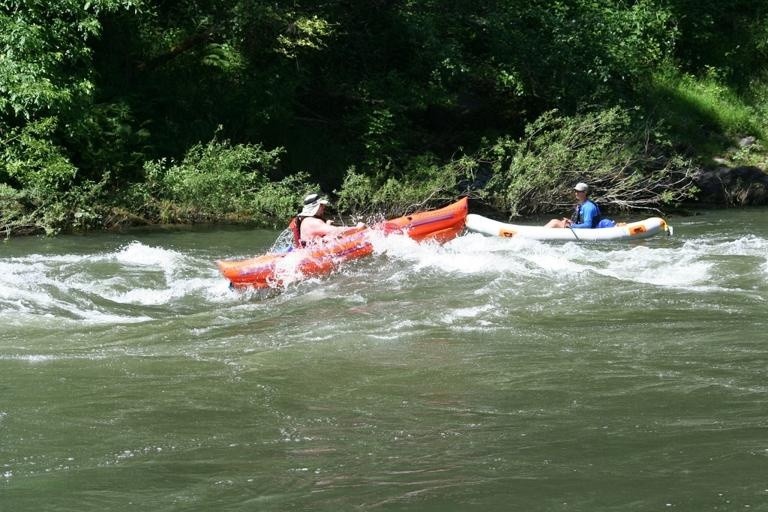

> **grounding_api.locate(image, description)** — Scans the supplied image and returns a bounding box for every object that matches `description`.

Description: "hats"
[297,194,328,216]
[573,183,588,191]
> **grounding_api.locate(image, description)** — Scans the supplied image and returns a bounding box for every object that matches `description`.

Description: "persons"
[289,193,369,248]
[541,184,602,231]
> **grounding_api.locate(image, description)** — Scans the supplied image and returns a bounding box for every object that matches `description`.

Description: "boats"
[463,210,671,244]
[212,193,470,290]
